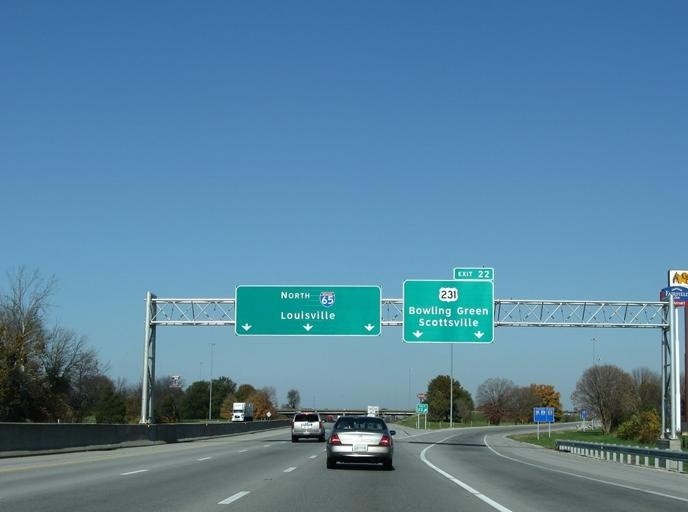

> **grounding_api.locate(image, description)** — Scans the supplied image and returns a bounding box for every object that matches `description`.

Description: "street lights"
[209,344,216,421]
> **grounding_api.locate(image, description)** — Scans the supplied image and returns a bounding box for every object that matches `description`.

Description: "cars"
[292,411,325,442]
[327,416,395,470]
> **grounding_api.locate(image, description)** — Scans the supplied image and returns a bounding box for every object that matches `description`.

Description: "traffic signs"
[234,279,494,343]
[416,404,428,413]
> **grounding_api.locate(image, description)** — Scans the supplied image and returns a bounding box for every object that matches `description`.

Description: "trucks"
[232,403,253,421]
